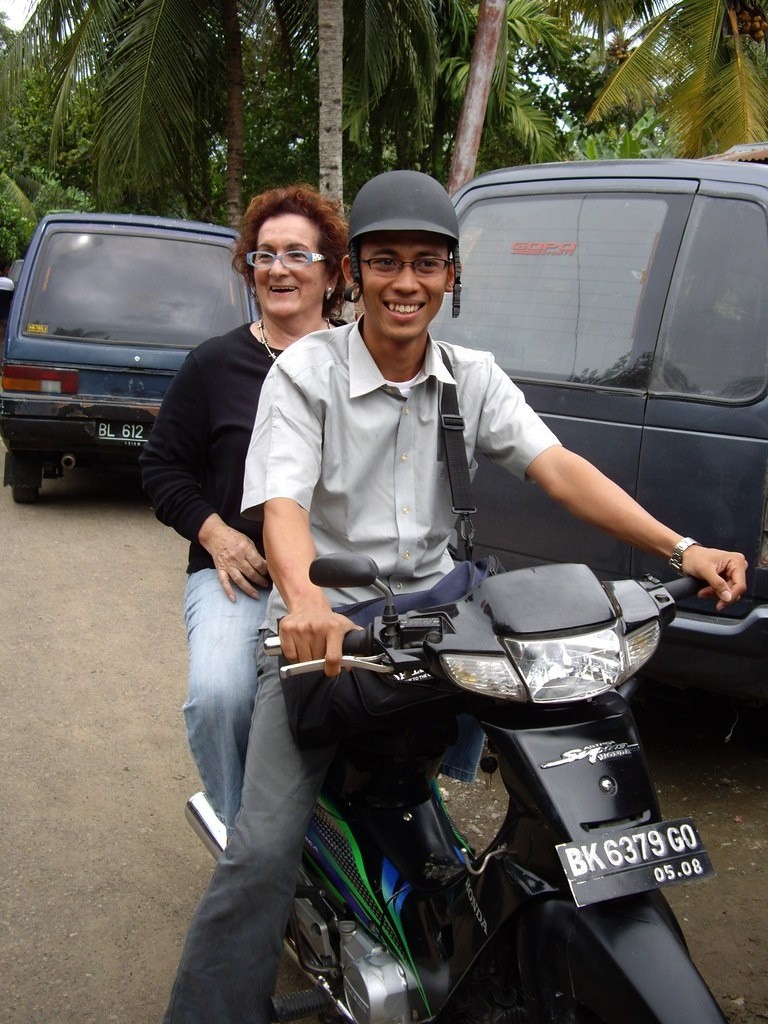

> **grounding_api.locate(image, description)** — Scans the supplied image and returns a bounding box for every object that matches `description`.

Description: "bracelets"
[669,535,702,579]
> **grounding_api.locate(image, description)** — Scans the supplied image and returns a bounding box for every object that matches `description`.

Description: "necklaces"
[258,317,331,361]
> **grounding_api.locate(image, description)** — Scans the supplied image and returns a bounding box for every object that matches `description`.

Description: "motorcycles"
[180,549,767,1024]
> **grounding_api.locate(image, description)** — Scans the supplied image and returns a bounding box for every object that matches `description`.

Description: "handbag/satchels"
[276,555,508,748]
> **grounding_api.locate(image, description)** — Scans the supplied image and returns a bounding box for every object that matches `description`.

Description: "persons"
[140,180,354,834]
[164,170,748,1024]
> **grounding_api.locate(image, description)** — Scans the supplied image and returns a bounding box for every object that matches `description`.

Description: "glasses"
[356,256,451,278]
[243,250,326,270]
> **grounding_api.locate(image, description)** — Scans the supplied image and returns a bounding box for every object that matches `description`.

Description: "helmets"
[348,171,459,248]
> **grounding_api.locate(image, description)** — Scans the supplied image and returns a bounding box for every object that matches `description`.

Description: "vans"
[4,212,263,503]
[429,156,767,707]
